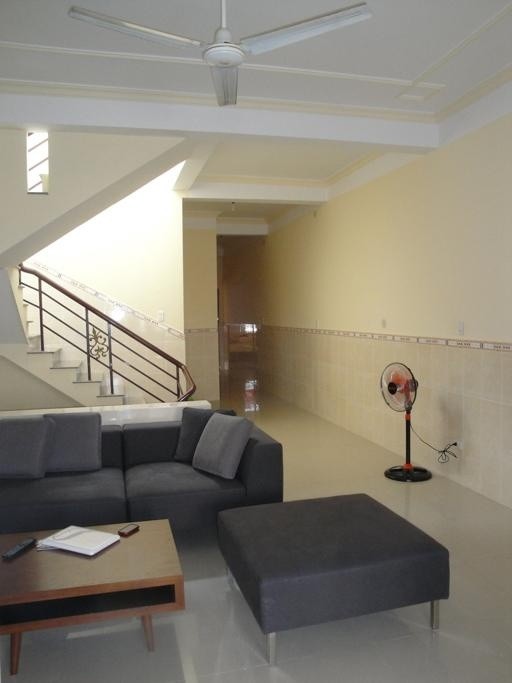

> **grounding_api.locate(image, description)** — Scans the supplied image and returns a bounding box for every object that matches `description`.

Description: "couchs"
[1,413,280,544]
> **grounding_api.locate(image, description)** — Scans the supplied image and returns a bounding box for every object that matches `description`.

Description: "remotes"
[2,536,36,562]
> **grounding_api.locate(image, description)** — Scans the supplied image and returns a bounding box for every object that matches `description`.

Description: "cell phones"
[117,523,140,537]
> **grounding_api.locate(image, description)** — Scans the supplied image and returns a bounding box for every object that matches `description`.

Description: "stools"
[218,494,450,667]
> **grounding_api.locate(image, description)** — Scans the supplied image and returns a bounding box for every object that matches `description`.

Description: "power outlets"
[453,439,464,450]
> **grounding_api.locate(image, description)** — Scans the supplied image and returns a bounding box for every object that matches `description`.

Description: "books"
[40,525,121,557]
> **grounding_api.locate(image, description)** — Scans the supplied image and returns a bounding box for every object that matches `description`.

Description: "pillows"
[194,412,252,479]
[0,417,48,480]
[177,407,235,461]
[45,411,102,475]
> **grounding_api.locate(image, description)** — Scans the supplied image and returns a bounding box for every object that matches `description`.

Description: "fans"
[65,3,378,106]
[381,363,432,481]
